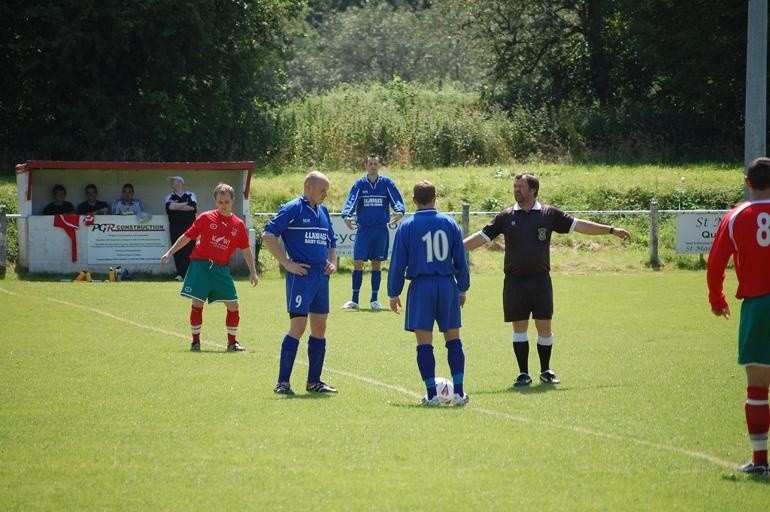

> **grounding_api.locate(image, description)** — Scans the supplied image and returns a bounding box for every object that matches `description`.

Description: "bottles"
[109,267,115,282]
[85,271,92,282]
[77,271,86,281]
[116,266,122,281]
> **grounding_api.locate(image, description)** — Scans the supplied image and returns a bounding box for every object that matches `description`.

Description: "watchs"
[609,226,614,234]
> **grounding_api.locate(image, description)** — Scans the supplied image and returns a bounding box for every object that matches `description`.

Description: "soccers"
[434,376,454,404]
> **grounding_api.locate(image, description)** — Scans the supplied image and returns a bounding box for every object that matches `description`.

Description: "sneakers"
[189,341,201,351]
[273,383,294,395]
[341,300,359,310]
[369,300,383,309]
[538,369,561,385]
[305,379,339,394]
[174,274,183,282]
[512,372,532,386]
[738,458,770,479]
[227,340,245,351]
[420,394,441,406]
[450,392,470,407]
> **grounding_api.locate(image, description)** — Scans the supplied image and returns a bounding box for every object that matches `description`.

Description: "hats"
[166,175,186,185]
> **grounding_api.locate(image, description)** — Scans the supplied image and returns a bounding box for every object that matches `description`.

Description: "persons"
[77,184,111,215]
[262,171,338,394]
[463,172,631,386]
[342,153,405,312]
[706,158,769,473]
[161,184,258,352]
[43,184,74,215]
[387,180,470,406]
[164,176,197,281]
[112,183,143,215]
